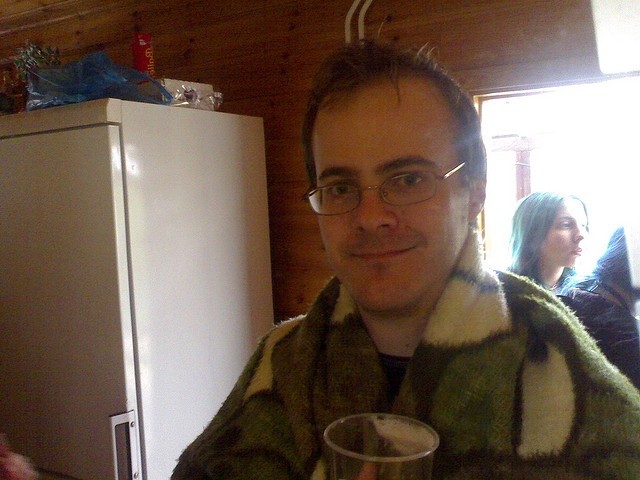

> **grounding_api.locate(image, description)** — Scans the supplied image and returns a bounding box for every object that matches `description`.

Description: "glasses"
[301,160,469,216]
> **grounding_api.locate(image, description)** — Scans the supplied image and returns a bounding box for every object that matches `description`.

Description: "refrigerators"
[0,96,276,480]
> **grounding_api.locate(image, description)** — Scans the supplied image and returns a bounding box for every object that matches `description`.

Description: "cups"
[320,411,442,480]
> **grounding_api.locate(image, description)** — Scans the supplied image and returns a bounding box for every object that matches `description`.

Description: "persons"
[505,191,589,302]
[169,39,640,480]
[557,226,640,391]
